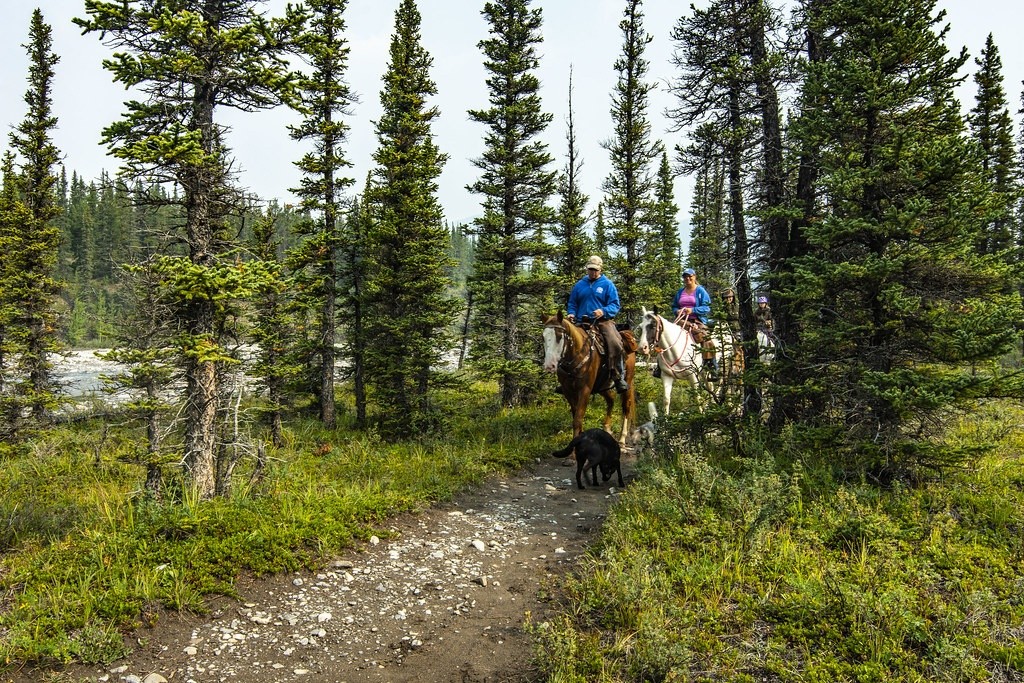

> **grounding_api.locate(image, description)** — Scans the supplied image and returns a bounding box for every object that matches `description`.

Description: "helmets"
[757,297,767,304]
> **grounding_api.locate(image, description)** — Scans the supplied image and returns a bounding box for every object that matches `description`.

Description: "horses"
[635,306,775,417]
[538,310,638,453]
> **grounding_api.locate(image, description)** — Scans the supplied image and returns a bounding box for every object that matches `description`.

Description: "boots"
[707,356,720,380]
[555,387,563,393]
[615,354,627,394]
[653,364,660,377]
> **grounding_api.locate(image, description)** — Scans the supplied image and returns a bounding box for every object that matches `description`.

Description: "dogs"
[629,402,658,460]
[552,428,626,489]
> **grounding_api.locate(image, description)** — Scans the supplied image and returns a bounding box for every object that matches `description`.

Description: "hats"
[682,269,695,276]
[724,289,733,297]
[587,255,602,270]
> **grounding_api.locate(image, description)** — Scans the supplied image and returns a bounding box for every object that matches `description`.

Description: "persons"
[722,288,776,387]
[555,255,629,393]
[653,267,718,381]
